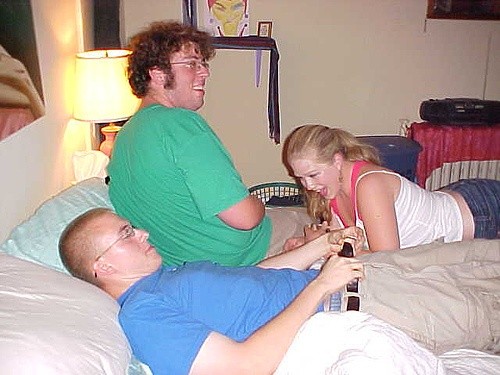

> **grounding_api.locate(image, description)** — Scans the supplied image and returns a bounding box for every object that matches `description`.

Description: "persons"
[106,22,321,268]
[282,124,500,254]
[59,209,500,375]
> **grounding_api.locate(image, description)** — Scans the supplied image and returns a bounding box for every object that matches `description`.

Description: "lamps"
[72,51,138,160]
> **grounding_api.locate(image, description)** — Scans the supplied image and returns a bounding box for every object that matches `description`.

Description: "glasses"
[93,224,136,278]
[169,61,210,71]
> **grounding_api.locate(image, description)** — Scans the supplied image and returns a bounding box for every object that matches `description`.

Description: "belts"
[338,242,359,312]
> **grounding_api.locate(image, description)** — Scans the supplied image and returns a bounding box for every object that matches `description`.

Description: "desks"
[405,121,500,193]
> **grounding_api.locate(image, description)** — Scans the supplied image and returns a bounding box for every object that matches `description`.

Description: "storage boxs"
[348,136,422,185]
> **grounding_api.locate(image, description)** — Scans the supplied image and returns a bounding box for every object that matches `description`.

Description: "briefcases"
[420,98,500,125]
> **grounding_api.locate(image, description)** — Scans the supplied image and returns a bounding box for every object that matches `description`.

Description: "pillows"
[0,251,135,375]
[0,177,119,276]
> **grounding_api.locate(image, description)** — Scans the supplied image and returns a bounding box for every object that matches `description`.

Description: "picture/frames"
[258,22,272,38]
[427,0,500,21]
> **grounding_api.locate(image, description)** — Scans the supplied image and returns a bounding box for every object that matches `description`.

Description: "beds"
[0,111,499,374]
